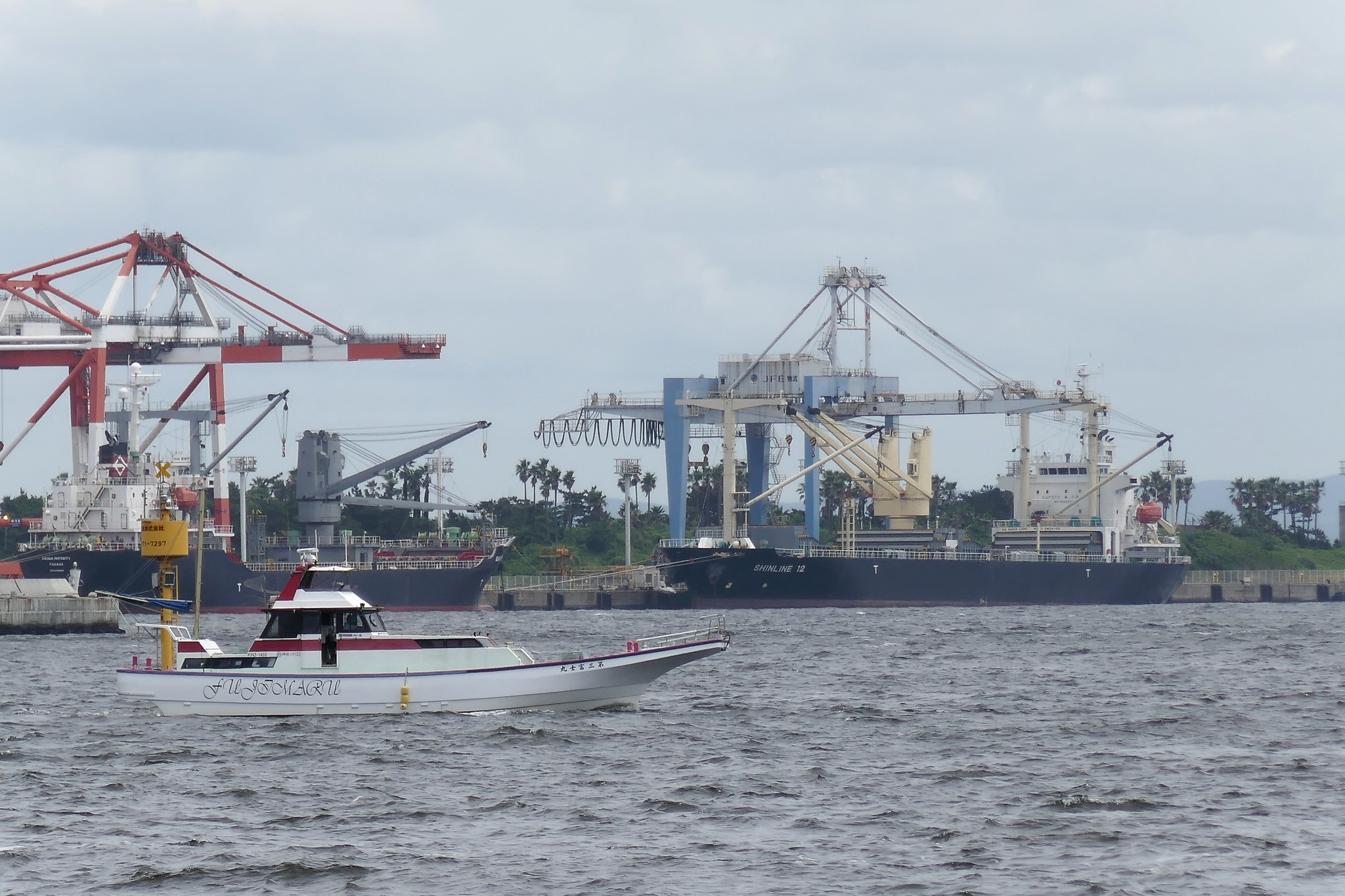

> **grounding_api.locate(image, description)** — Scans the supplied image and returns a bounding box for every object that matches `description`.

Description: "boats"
[115,530,730,716]
[0,452,518,614]
[650,363,1192,607]
[0,561,126,637]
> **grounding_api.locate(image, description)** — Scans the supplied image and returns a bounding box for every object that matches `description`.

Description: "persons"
[343,615,360,632]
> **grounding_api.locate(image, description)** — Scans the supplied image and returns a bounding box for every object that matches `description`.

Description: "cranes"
[533,255,1110,541]
[0,230,494,553]
